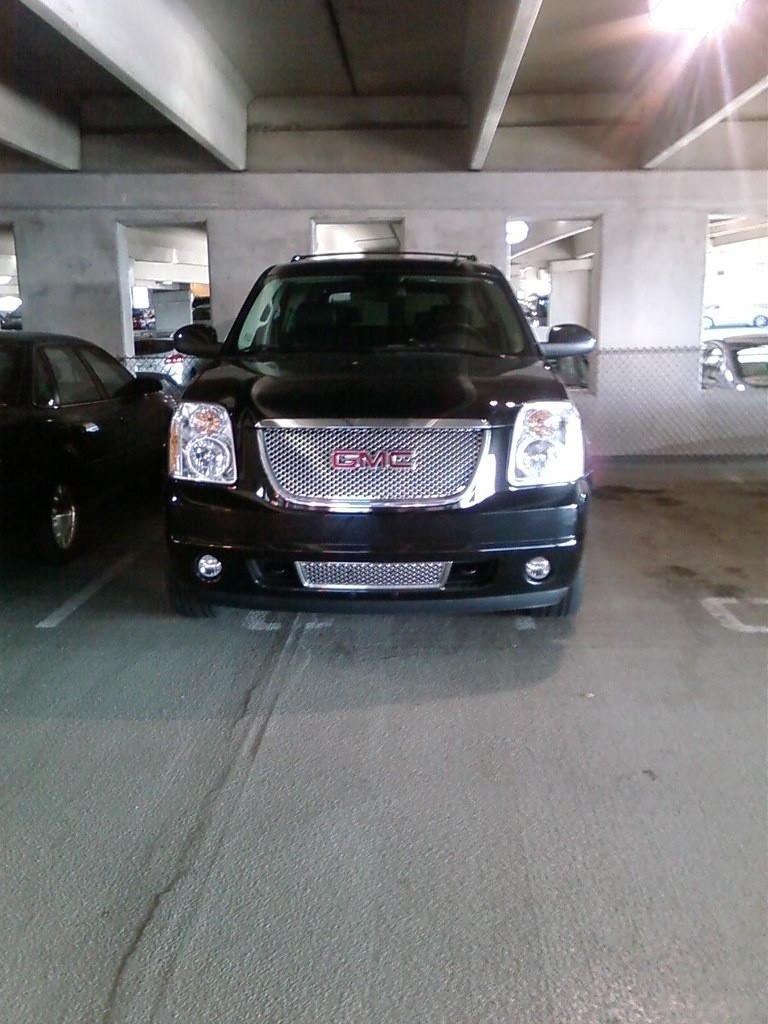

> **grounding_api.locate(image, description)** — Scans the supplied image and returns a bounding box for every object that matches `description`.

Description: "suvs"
[162,245,597,621]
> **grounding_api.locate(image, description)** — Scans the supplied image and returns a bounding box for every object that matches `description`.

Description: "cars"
[701,300,768,331]
[132,307,158,329]
[132,327,201,391]
[0,329,175,567]
[518,290,548,326]
[189,294,212,326]
[702,332,768,391]
[0,303,23,330]
